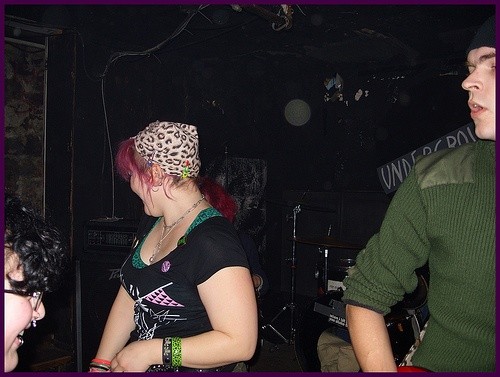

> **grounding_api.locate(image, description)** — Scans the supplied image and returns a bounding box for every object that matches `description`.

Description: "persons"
[343,15,496,372]
[5,195,66,372]
[90,122,258,372]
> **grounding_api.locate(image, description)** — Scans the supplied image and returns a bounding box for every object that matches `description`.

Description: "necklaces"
[148,195,205,262]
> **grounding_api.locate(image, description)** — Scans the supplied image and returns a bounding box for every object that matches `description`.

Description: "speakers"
[72,257,124,372]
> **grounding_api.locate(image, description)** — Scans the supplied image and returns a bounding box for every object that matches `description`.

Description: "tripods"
[259,205,301,345]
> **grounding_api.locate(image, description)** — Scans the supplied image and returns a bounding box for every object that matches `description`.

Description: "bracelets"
[89,358,111,372]
[162,336,172,366]
[172,337,182,368]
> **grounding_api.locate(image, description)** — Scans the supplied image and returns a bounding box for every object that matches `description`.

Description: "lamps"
[334,88,363,102]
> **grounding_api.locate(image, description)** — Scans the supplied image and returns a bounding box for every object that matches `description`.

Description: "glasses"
[5,289,44,312]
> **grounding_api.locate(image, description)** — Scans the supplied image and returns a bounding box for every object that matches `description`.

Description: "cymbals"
[288,235,365,250]
[270,201,337,212]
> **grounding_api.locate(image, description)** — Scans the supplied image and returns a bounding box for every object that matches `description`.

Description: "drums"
[294,289,344,372]
[316,258,355,299]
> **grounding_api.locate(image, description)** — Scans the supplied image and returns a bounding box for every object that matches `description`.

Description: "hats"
[132,121,202,177]
[465,15,496,61]
